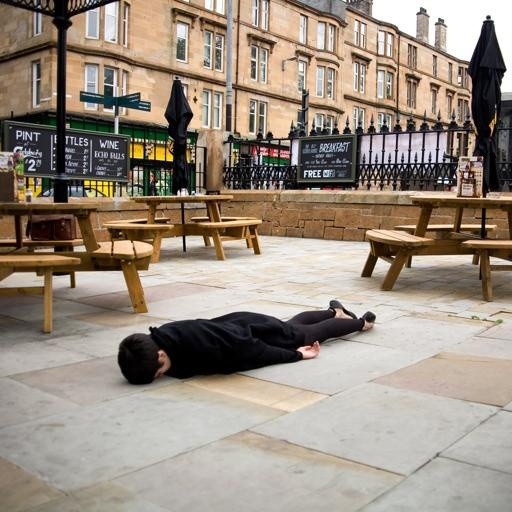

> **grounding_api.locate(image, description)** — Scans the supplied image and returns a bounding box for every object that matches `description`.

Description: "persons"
[13,148,22,169]
[116,300,377,386]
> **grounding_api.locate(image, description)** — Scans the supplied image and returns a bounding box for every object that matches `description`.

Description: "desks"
[0,194,234,270]
[409,194,511,237]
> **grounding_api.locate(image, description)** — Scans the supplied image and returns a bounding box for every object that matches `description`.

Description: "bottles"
[25,189,32,203]
[16,176,24,201]
[176,188,190,196]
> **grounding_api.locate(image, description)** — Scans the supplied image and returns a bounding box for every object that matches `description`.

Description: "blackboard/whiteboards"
[297,134,357,183]
[2,120,130,182]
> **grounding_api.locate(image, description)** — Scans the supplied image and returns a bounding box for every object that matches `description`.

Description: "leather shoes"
[329,300,357,320]
[360,311,376,322]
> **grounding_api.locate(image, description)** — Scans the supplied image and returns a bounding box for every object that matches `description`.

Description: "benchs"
[0,215,262,334]
[361,224,512,302]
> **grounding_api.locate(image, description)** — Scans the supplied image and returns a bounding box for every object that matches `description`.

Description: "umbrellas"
[163,75,194,250]
[466,14,509,282]
